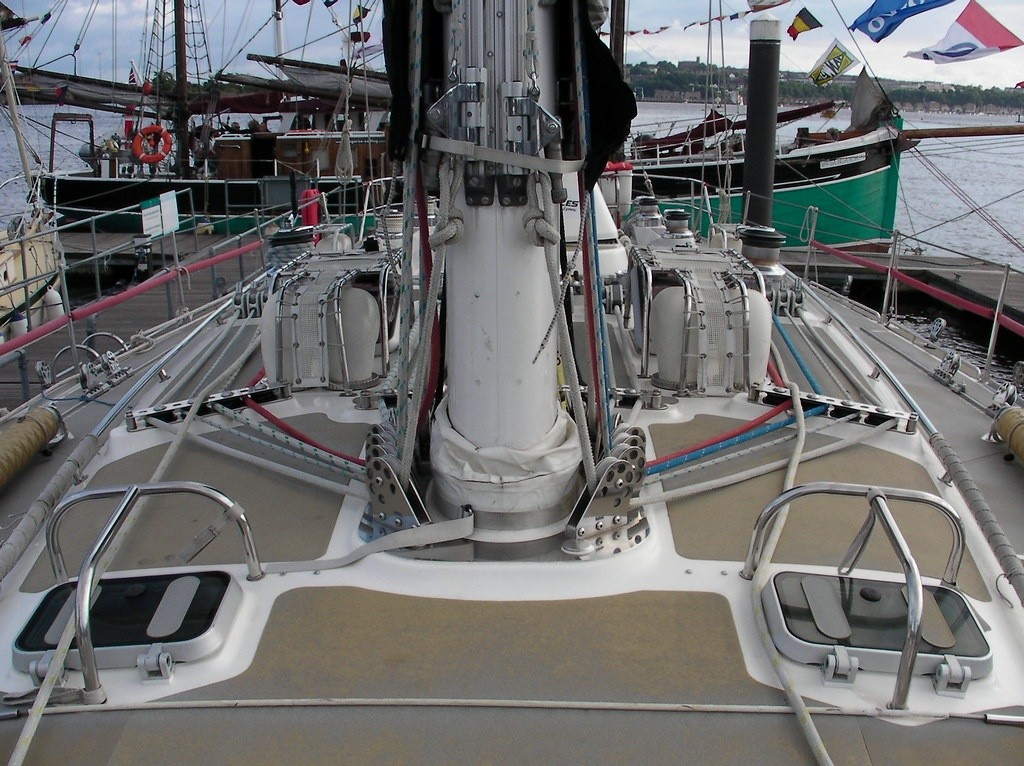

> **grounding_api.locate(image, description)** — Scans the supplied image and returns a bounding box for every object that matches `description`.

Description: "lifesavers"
[132,125,173,163]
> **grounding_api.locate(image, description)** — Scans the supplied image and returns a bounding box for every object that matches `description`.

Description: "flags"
[808,39,860,86]
[125,68,138,142]
[747,0,791,12]
[848,0,954,42]
[787,8,822,41]
[293,0,372,44]
[684,10,750,30]
[903,0,1024,65]
[56,87,66,106]
[600,26,669,36]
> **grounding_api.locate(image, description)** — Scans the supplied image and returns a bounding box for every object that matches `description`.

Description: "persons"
[112,132,121,150]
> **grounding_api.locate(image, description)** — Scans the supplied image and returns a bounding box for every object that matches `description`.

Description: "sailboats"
[0,0,920,253]
